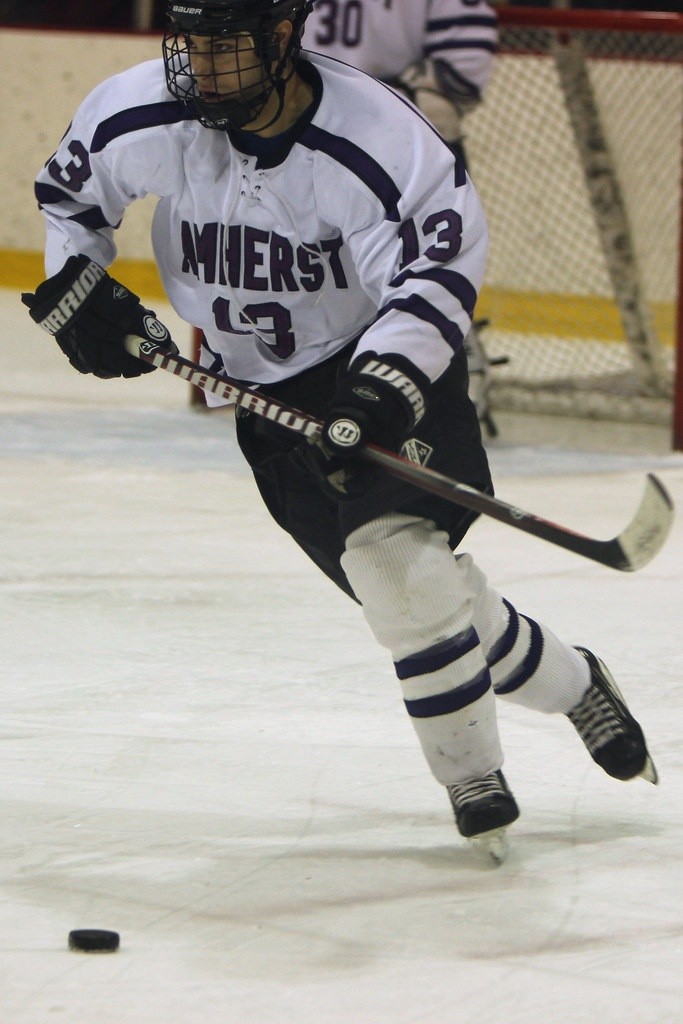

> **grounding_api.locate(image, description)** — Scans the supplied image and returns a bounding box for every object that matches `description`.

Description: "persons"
[21,0,660,864]
[299,0,510,438]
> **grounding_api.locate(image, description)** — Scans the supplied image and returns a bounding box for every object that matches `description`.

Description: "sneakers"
[563,645,664,782]
[444,769,521,863]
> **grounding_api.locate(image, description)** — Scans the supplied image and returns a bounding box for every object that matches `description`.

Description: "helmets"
[159,1,314,136]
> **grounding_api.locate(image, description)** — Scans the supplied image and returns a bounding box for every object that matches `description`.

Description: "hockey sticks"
[127,335,677,576]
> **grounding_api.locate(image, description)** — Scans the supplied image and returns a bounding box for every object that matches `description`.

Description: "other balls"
[67,927,121,954]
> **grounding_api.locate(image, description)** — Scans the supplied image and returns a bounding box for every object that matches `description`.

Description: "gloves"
[315,355,429,498]
[17,254,181,378]
[394,55,485,151]
